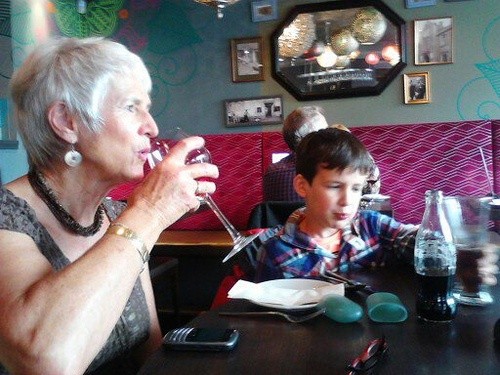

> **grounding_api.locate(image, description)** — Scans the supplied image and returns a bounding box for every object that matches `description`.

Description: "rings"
[195,180,199,193]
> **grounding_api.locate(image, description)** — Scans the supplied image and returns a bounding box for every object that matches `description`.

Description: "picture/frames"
[413,16,454,65]
[223,94,285,128]
[251,0,279,22]
[404,72,432,105]
[230,35,265,82]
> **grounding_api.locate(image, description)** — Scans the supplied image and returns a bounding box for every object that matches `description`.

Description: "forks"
[320,274,366,291]
[217,307,327,323]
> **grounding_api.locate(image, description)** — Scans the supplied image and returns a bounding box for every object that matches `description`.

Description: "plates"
[248,278,335,311]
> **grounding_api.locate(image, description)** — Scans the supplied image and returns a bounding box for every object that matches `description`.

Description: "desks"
[83,266,500,375]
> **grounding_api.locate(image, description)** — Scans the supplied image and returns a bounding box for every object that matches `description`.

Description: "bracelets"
[106,223,151,263]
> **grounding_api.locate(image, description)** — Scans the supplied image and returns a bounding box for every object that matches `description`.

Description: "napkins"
[227,278,345,307]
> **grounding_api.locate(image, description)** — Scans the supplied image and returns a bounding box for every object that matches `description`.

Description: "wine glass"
[443,196,495,307]
[145,129,266,263]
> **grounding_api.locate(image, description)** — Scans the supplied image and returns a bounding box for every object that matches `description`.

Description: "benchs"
[106,119,500,310]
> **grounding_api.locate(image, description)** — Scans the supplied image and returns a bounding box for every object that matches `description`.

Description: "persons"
[210,128,498,310]
[0,38,220,375]
[409,80,425,100]
[261,107,381,203]
[242,109,249,122]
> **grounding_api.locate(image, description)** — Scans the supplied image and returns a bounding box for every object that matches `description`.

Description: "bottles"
[413,188,458,324]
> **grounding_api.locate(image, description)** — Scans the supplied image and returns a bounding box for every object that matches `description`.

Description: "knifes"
[326,270,377,294]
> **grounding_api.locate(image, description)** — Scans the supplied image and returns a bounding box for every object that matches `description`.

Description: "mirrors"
[272,0,406,102]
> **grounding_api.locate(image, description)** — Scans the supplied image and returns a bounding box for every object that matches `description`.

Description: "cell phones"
[161,328,239,351]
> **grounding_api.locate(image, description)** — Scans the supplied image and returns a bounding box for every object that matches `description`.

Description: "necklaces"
[28,166,104,237]
